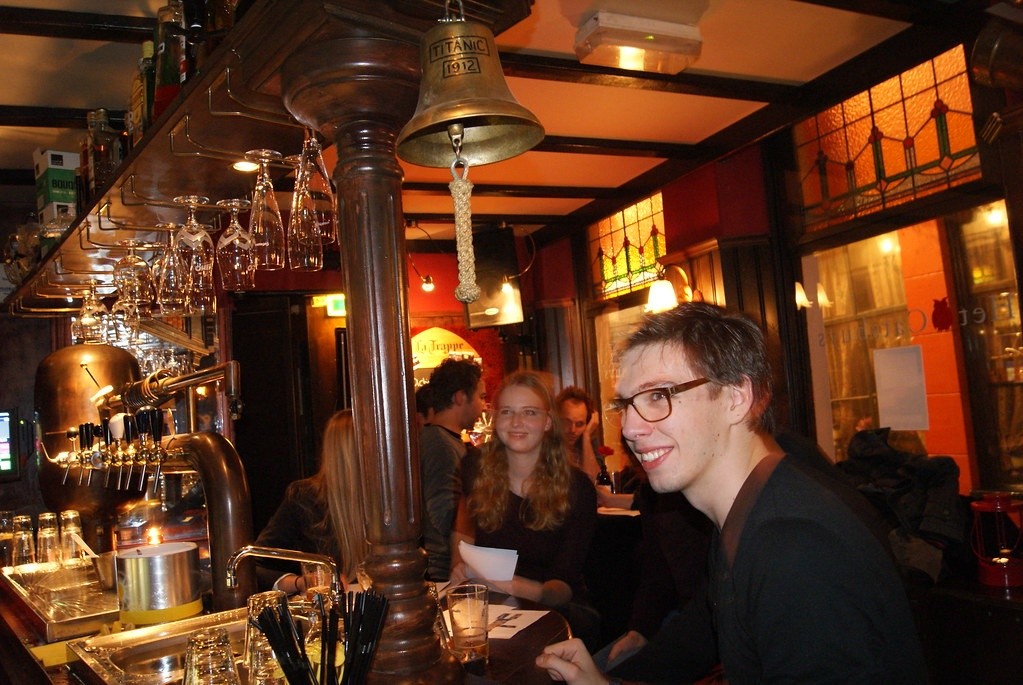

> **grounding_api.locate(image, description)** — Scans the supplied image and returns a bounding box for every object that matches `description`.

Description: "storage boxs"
[33,147,81,261]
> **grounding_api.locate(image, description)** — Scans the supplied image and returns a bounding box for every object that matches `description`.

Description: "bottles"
[73,0,254,214]
[597,466,614,494]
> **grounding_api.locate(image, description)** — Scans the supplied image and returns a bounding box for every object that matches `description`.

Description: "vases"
[596,466,614,494]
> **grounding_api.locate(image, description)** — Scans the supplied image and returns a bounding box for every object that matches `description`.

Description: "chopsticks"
[250,589,391,685]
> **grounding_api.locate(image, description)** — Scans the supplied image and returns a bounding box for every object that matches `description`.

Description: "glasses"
[604,372,733,428]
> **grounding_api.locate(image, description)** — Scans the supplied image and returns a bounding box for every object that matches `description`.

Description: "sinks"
[109,615,316,672]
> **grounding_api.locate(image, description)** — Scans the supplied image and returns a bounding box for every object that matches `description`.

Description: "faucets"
[61,405,185,494]
[225,546,346,617]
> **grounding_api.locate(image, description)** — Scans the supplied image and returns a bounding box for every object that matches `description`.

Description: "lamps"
[498,221,536,293]
[969,493,1023,587]
[645,265,703,315]
[571,12,702,76]
[403,220,435,291]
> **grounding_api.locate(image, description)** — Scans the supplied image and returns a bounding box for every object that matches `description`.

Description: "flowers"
[598,444,615,465]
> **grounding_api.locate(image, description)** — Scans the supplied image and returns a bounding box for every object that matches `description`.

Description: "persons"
[250,301,1021,685]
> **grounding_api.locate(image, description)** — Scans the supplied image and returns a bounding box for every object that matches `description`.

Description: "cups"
[11,509,85,574]
[182,591,345,685]
[302,556,336,610]
[446,584,490,661]
[90,551,117,590]
[355,561,372,591]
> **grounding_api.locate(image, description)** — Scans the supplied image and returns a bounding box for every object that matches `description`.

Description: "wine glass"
[70,128,335,345]
